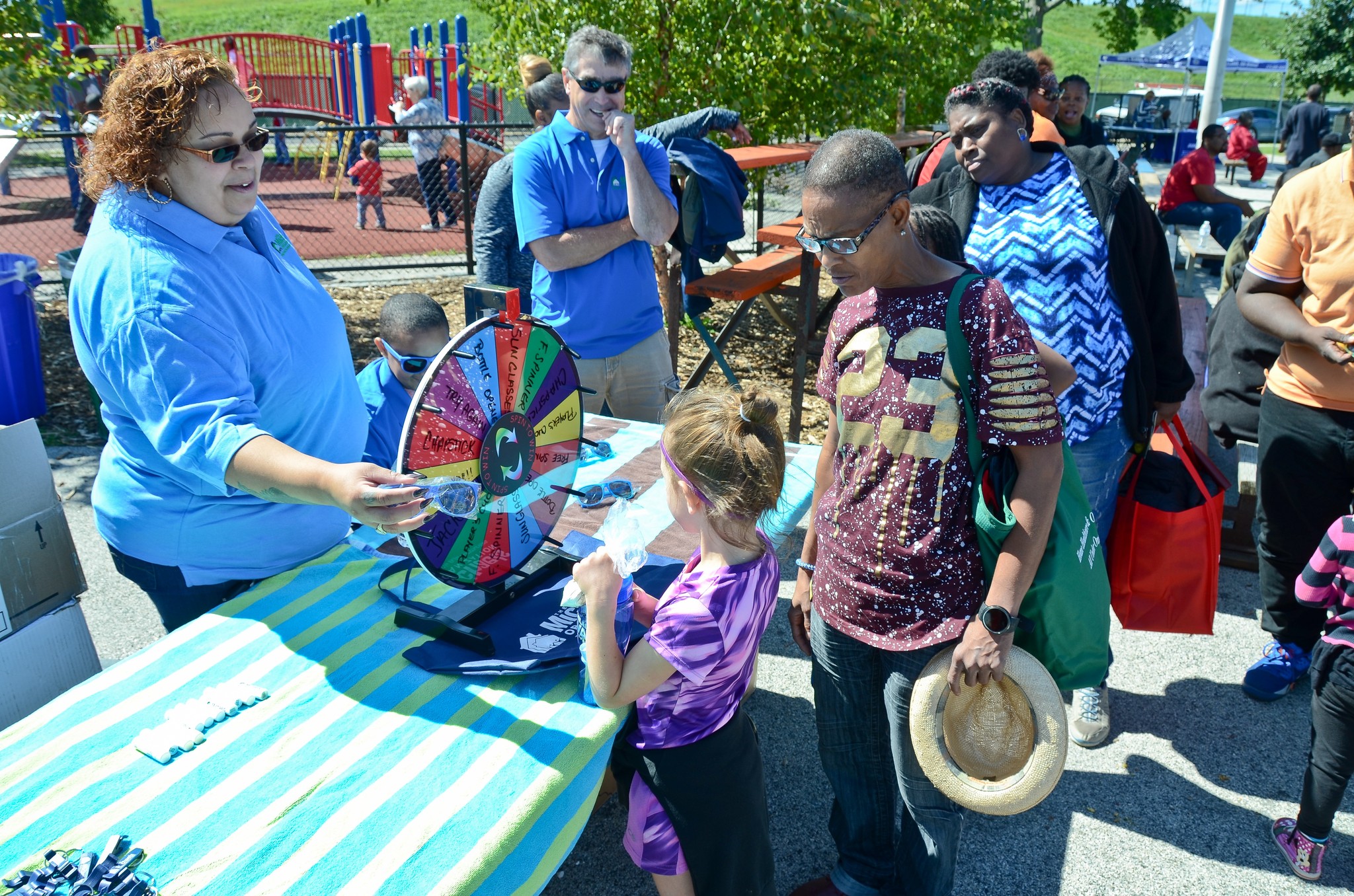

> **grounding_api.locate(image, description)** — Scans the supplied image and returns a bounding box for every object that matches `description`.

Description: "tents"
[1093,17,1289,172]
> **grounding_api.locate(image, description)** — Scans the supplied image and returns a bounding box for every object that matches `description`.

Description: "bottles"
[1198,221,1212,249]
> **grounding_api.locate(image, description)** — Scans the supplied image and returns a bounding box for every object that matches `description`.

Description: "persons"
[59,38,432,636]
[349,23,1354,896]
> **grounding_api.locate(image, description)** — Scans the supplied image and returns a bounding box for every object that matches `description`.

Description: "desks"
[0,402,826,896]
[1140,172,1165,213]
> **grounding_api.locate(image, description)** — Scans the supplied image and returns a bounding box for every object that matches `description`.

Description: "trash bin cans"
[56,248,110,440]
[0,252,46,426]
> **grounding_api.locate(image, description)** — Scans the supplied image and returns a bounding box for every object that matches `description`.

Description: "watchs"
[978,601,1020,634]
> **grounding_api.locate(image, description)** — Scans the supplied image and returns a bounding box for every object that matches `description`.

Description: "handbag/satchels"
[377,550,636,676]
[1109,413,1230,636]
[943,274,1111,704]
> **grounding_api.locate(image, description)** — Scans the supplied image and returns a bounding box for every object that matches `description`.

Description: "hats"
[910,644,1068,815]
[1322,132,1344,146]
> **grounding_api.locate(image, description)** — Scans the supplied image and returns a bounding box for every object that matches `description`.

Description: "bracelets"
[795,558,818,570]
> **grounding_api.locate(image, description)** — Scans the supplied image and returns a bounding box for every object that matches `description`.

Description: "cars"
[1215,105,1287,144]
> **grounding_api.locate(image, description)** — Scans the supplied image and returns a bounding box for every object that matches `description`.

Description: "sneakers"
[1068,681,1111,746]
[1241,640,1309,700]
[1272,817,1334,880]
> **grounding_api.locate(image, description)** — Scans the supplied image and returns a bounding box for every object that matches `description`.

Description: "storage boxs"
[0,247,104,730]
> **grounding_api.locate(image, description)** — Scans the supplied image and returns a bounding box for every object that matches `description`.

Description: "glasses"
[172,126,269,164]
[578,441,613,466]
[567,68,627,94]
[380,482,482,519]
[572,480,637,508]
[382,337,454,374]
[1212,134,1229,140]
[1036,86,1065,101]
[795,190,912,254]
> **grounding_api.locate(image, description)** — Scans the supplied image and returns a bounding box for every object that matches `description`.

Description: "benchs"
[684,238,826,391]
[1163,223,1227,297]
[1218,153,1248,186]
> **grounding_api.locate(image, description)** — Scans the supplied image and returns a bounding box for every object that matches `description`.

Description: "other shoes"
[440,220,458,228]
[74,223,91,235]
[355,223,365,230]
[421,223,440,232]
[789,875,850,896]
[376,225,386,230]
[1202,260,1221,277]
